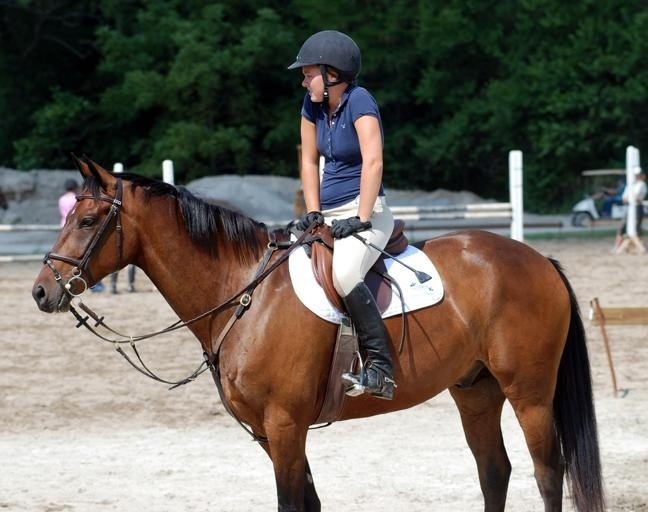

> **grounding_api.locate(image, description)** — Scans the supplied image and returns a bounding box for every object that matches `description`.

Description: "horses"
[30,153,607,512]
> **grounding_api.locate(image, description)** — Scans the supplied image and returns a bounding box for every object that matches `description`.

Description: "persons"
[110,261,138,295]
[287,29,399,401]
[600,176,625,215]
[612,164,648,257]
[58,179,104,292]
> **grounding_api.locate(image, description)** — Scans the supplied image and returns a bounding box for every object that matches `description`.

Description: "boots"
[339,280,396,402]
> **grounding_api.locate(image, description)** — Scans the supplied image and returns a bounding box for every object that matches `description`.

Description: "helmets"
[285,28,362,84]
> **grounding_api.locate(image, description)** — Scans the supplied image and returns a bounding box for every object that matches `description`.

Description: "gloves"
[295,210,325,232]
[329,216,373,239]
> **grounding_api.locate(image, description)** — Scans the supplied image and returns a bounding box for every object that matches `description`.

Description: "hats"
[63,177,77,191]
